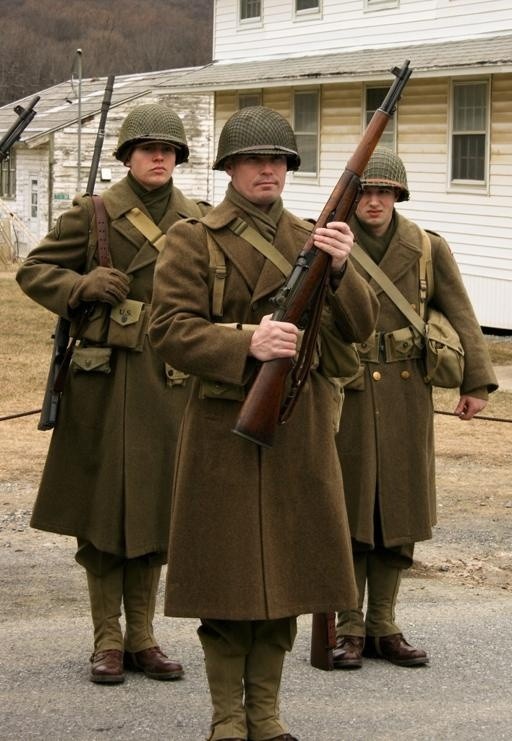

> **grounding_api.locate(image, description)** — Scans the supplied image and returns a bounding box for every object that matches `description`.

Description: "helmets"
[112,104,190,167]
[353,145,409,203]
[211,105,301,172]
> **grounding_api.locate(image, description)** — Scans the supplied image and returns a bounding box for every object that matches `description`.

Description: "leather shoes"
[332,632,366,670]
[215,734,300,741]
[125,643,186,681]
[89,644,126,684]
[366,629,431,668]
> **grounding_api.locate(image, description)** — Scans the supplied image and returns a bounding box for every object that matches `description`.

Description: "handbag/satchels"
[327,377,345,435]
[421,305,468,388]
[199,322,262,404]
[69,292,191,388]
[316,302,360,380]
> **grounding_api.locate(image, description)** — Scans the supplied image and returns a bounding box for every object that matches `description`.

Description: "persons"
[309,145,497,669]
[145,106,381,741]
[15,103,217,686]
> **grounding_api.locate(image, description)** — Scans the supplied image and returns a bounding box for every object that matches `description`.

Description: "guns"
[36,74,116,431]
[231,58,413,452]
[0,94,43,163]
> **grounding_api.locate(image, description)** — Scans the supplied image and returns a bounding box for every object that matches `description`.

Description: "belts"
[69,267,131,310]
